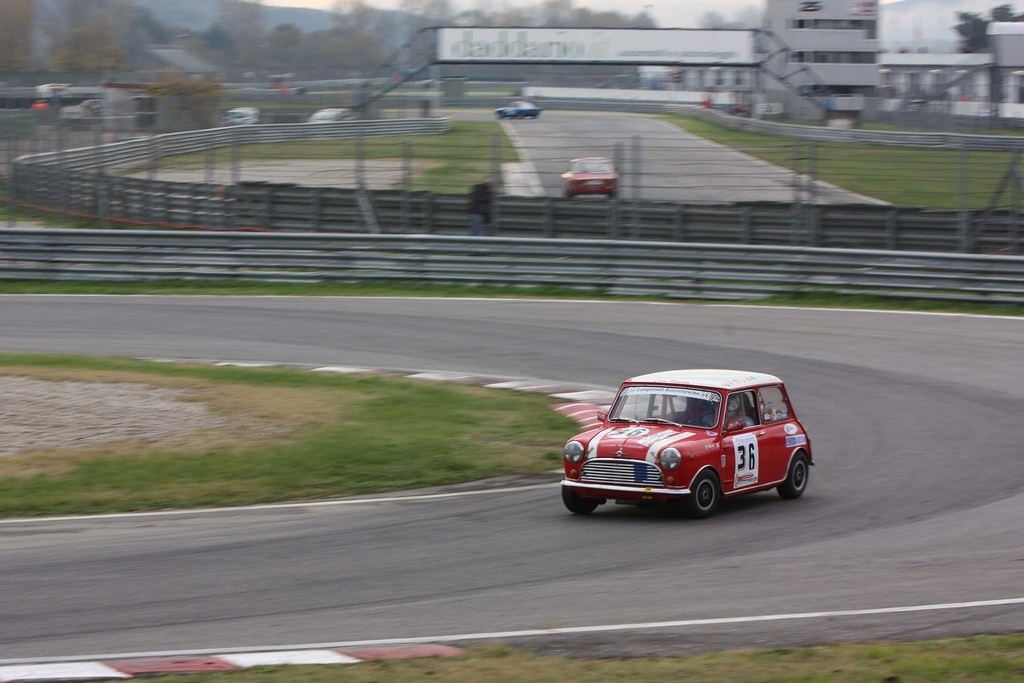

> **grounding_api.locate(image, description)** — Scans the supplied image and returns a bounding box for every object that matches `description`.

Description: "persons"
[701,395,754,430]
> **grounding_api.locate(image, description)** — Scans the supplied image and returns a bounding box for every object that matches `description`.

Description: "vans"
[308,109,356,123]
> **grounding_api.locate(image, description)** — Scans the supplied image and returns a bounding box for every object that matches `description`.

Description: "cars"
[56,98,106,122]
[561,157,622,200]
[491,100,543,119]
[559,368,815,519]
[224,107,257,125]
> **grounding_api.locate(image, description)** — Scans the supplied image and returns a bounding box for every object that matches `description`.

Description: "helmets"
[710,396,740,411]
